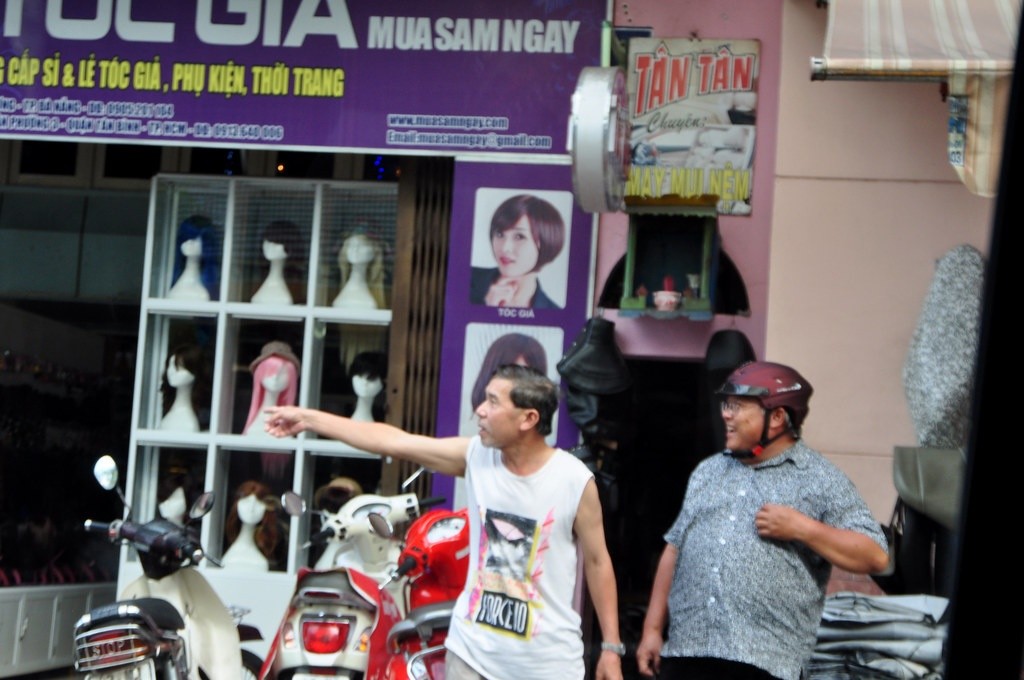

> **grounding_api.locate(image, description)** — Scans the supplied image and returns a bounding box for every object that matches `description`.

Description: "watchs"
[601,642,626,655]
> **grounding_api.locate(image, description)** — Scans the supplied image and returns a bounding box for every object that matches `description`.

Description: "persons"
[251,222,306,305]
[220,482,277,569]
[245,343,303,436]
[156,349,207,433]
[470,331,548,420]
[346,354,389,423]
[165,216,213,299]
[313,480,359,569]
[157,474,194,528]
[469,194,565,308]
[265,364,627,680]
[333,230,388,308]
[637,363,889,680]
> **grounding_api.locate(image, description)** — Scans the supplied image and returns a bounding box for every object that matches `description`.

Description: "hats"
[248,342,300,377]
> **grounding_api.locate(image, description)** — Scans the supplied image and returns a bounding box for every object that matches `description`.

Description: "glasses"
[719,400,764,415]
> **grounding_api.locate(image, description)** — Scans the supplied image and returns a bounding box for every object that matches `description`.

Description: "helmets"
[716,362,814,427]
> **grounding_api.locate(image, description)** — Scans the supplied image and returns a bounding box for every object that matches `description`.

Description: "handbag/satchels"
[870,497,933,595]
[892,430,966,531]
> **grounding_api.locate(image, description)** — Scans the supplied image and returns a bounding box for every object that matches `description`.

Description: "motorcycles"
[72,452,242,680]
[253,460,472,680]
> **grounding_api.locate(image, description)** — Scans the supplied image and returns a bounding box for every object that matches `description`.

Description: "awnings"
[810,0,1024,196]
